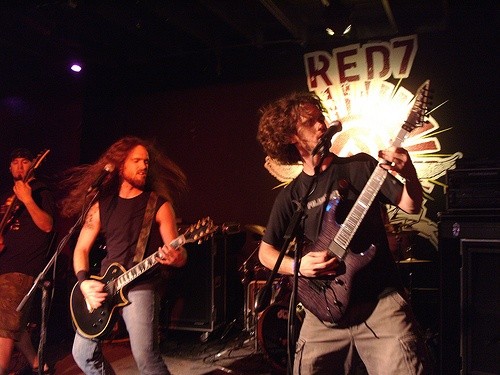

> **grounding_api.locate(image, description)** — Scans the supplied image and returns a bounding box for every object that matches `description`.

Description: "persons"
[0,147,59,375]
[258,91,424,375]
[55,136,187,375]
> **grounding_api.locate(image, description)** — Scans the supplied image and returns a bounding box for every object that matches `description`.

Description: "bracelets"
[77,271,89,286]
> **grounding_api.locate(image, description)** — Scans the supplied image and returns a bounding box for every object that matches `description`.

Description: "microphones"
[311,120,342,156]
[86,161,115,196]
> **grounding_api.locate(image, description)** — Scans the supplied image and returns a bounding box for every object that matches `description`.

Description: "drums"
[257,301,302,371]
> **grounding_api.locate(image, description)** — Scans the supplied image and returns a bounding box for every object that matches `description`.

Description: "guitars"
[287,80,434,327]
[69,217,218,340]
[0,147,49,237]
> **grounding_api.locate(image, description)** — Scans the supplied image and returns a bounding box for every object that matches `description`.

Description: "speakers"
[432,219,500,375]
